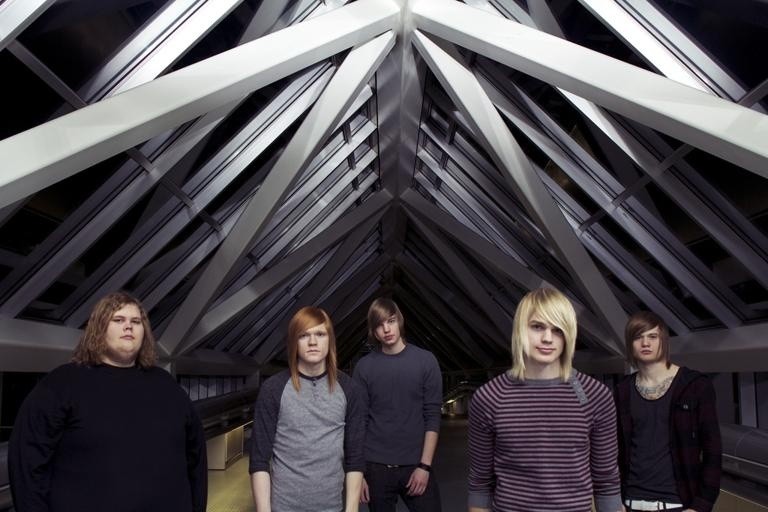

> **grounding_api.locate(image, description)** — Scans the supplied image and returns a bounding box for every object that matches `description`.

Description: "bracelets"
[415,462,432,472]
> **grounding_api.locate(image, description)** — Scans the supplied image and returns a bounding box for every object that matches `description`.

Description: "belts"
[624,500,684,511]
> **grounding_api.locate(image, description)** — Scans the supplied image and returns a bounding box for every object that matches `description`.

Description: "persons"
[247,307,368,512]
[613,309,721,511]
[463,288,625,511]
[8,291,208,512]
[352,298,444,512]
